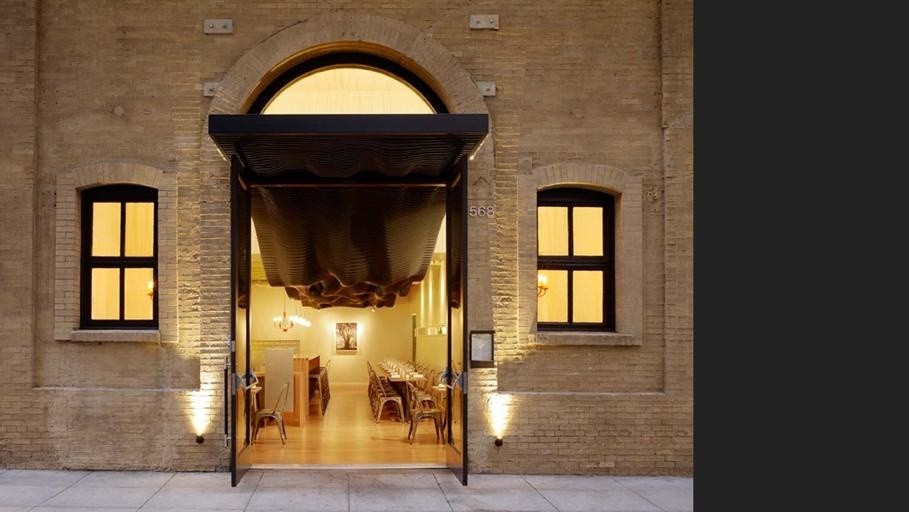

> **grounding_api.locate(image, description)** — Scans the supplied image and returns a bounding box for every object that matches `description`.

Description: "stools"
[310,366,330,415]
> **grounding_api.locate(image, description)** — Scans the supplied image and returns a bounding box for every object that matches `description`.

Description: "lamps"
[538,274,549,299]
[274,287,312,332]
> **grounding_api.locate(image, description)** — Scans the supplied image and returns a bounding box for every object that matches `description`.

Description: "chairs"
[367,359,446,445]
[250,382,289,447]
